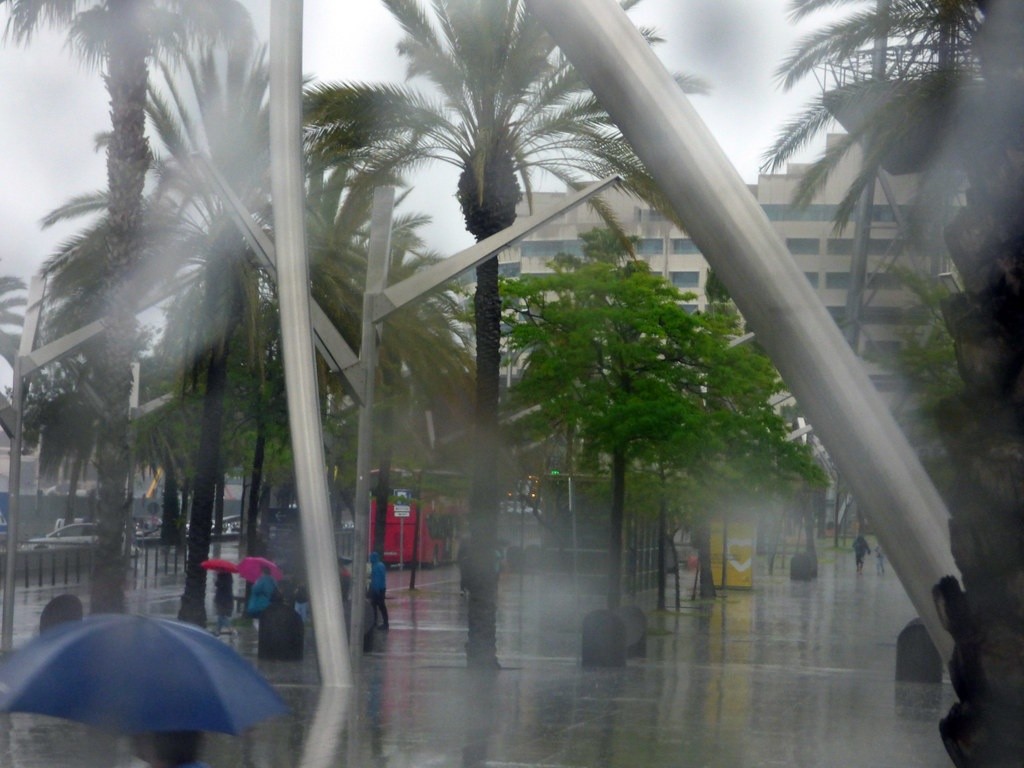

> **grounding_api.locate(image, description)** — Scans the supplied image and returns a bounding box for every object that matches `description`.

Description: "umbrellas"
[196,558,239,573]
[495,549,501,562]
[280,568,305,592]
[235,557,283,583]
[454,532,471,541]
[1,601,292,735]
[334,556,353,562]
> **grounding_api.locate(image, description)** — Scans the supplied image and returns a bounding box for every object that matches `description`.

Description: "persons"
[493,561,499,591]
[291,589,308,622]
[362,550,390,629]
[244,568,280,621]
[852,535,874,573]
[212,572,239,642]
[453,539,472,596]
[341,567,351,604]
[118,729,218,767]
[500,557,510,589]
[874,545,885,575]
